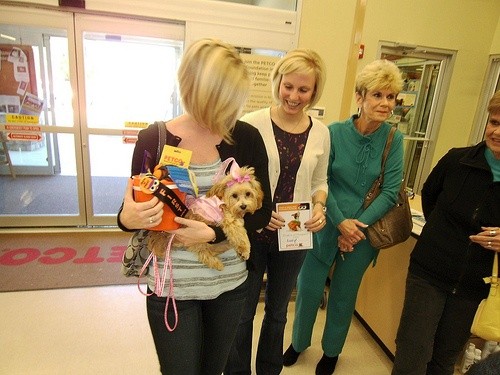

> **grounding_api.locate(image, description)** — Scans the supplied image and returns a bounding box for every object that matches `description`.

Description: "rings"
[320,219,323,224]
[488,242,491,247]
[149,216,153,223]
[490,230,496,236]
[351,238,354,242]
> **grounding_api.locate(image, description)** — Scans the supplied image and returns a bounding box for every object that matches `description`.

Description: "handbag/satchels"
[470,250,500,342]
[364,127,413,250]
[120,121,165,278]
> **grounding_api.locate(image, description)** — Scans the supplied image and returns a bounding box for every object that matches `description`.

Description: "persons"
[240,48,330,375]
[391,90,500,375]
[116,38,272,375]
[283,59,404,375]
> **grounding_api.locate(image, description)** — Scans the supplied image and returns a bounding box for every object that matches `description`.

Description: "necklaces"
[276,107,304,133]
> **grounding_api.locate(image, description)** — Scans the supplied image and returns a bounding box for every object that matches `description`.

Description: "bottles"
[459,346,475,374]
[474,349,481,362]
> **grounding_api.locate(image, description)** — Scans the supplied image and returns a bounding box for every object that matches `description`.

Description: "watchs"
[313,201,328,214]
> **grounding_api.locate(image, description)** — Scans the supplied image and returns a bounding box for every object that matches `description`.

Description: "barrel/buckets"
[132,175,187,230]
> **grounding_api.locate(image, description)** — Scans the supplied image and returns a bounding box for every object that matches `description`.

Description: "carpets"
[0,232,149,293]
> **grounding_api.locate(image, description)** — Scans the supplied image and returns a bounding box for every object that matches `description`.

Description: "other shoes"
[282,343,301,367]
[316,354,338,375]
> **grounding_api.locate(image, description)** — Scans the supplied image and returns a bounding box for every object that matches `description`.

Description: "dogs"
[149,166,264,272]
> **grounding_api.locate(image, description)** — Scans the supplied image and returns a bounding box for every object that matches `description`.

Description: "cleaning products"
[462,343,475,373]
[473,349,481,364]
[481,341,500,360]
